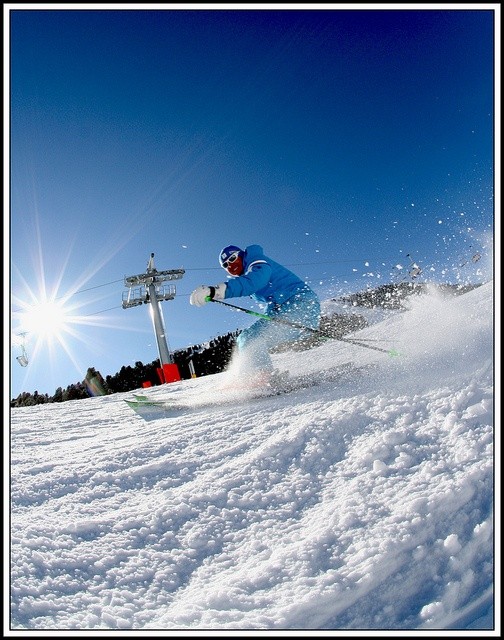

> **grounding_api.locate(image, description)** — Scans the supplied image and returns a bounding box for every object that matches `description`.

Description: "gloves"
[189,284,227,309]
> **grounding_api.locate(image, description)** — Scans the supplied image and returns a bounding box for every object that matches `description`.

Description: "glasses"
[221,251,241,270]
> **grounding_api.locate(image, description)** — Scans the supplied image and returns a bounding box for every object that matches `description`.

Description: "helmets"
[219,246,244,271]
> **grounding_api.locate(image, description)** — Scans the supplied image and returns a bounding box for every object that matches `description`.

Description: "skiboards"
[123,361,379,415]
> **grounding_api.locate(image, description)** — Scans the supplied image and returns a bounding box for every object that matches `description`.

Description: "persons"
[189,244,323,387]
